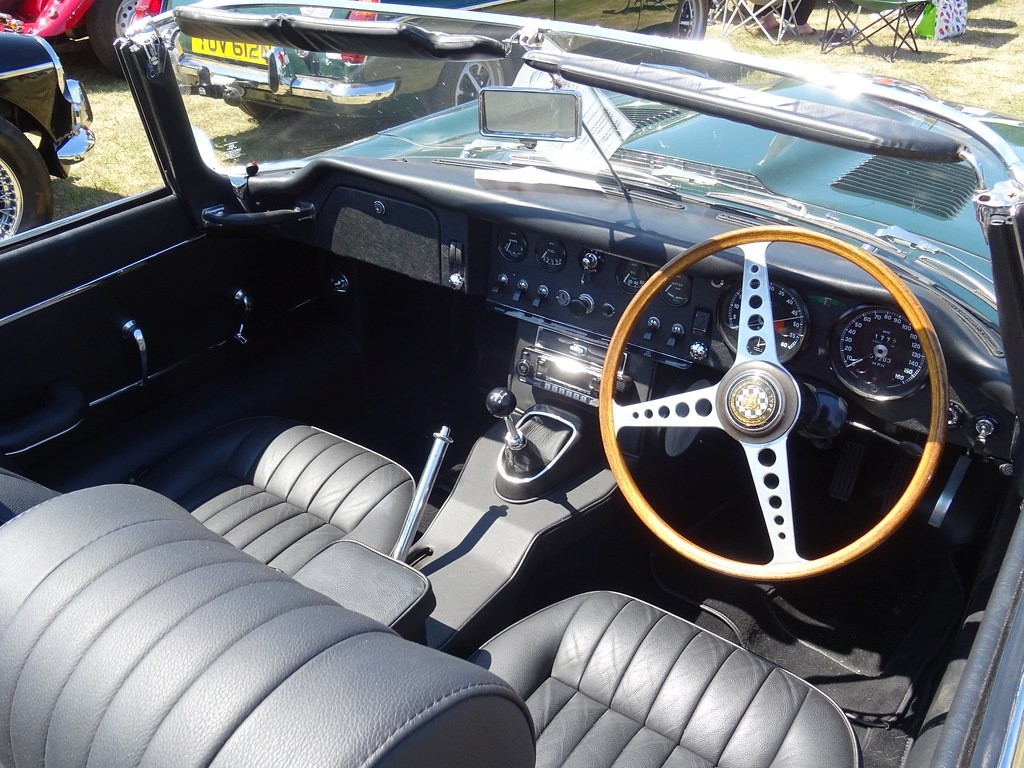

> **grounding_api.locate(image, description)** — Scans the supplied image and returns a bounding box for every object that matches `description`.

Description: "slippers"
[793,28,818,36]
[761,22,784,30]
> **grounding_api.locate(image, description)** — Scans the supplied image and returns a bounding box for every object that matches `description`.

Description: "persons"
[752,0,816,36]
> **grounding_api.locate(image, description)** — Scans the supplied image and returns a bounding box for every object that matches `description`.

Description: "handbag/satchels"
[914,0,967,39]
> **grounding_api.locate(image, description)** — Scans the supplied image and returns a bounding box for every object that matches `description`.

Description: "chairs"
[1,408,863,768]
[708,0,933,61]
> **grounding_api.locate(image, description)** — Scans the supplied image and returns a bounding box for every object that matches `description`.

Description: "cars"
[0,0,164,79]
[153,0,525,124]
